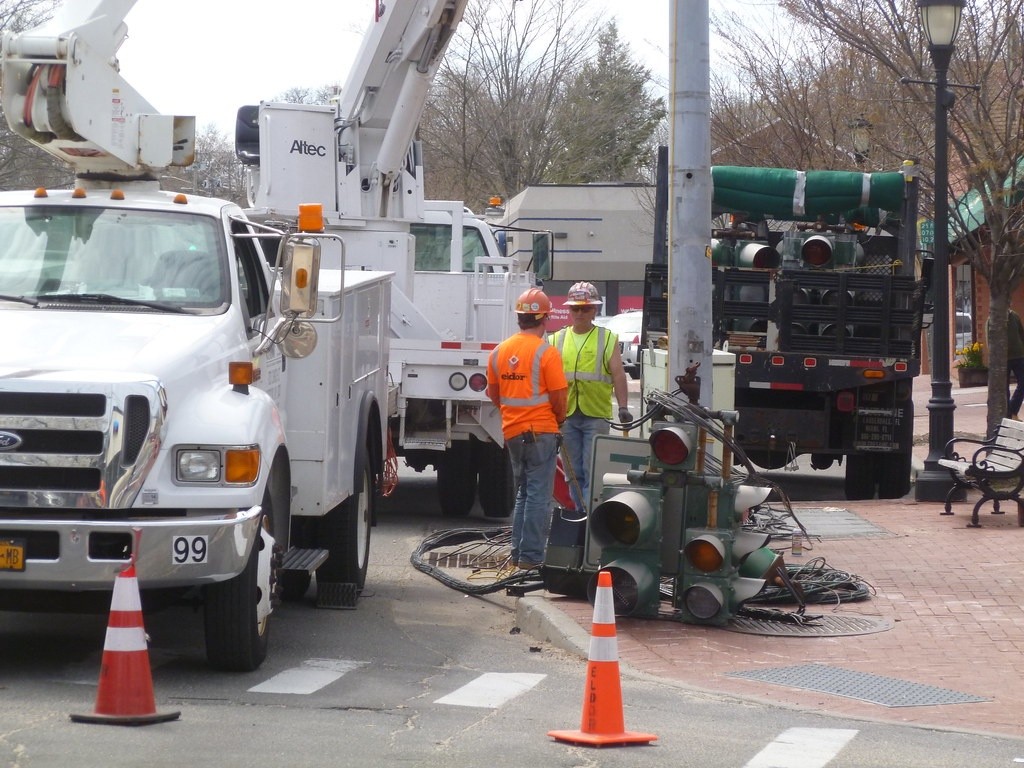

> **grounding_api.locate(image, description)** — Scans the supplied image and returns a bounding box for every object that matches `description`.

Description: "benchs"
[938,417,1024,527]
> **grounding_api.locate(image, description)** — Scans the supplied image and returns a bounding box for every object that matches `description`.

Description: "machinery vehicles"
[0,0,394,671]
[232,1,558,515]
[482,182,661,376]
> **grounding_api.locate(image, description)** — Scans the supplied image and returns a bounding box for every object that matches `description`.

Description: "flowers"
[952,341,986,368]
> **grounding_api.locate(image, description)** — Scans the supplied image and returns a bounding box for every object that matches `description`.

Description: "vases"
[958,368,989,388]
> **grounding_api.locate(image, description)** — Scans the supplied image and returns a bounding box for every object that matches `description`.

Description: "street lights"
[846,110,875,172]
[913,1,971,503]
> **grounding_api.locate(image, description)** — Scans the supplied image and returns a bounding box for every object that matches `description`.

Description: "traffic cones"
[545,567,660,749]
[67,549,181,726]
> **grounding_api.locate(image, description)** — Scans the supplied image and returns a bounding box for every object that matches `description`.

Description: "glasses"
[570,305,597,313]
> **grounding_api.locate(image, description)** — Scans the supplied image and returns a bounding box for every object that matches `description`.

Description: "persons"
[545,281,633,513]
[488,288,568,569]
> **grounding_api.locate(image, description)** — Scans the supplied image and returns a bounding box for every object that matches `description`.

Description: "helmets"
[514,288,551,313]
[562,281,603,306]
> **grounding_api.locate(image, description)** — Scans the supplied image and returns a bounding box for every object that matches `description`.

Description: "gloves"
[618,407,633,431]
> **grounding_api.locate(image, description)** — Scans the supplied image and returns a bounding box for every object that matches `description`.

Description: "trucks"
[646,143,939,499]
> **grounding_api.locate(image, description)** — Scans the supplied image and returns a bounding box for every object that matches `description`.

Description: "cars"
[954,310,971,362]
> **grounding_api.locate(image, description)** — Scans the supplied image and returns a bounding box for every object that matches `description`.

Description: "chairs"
[74,223,129,289]
[147,249,215,298]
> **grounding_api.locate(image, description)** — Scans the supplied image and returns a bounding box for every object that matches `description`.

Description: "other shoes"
[514,567,544,584]
[1012,414,1024,421]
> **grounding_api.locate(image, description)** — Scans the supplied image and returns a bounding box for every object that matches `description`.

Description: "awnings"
[946,150,1024,246]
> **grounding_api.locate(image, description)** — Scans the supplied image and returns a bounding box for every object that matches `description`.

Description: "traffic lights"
[587,428,780,627]
